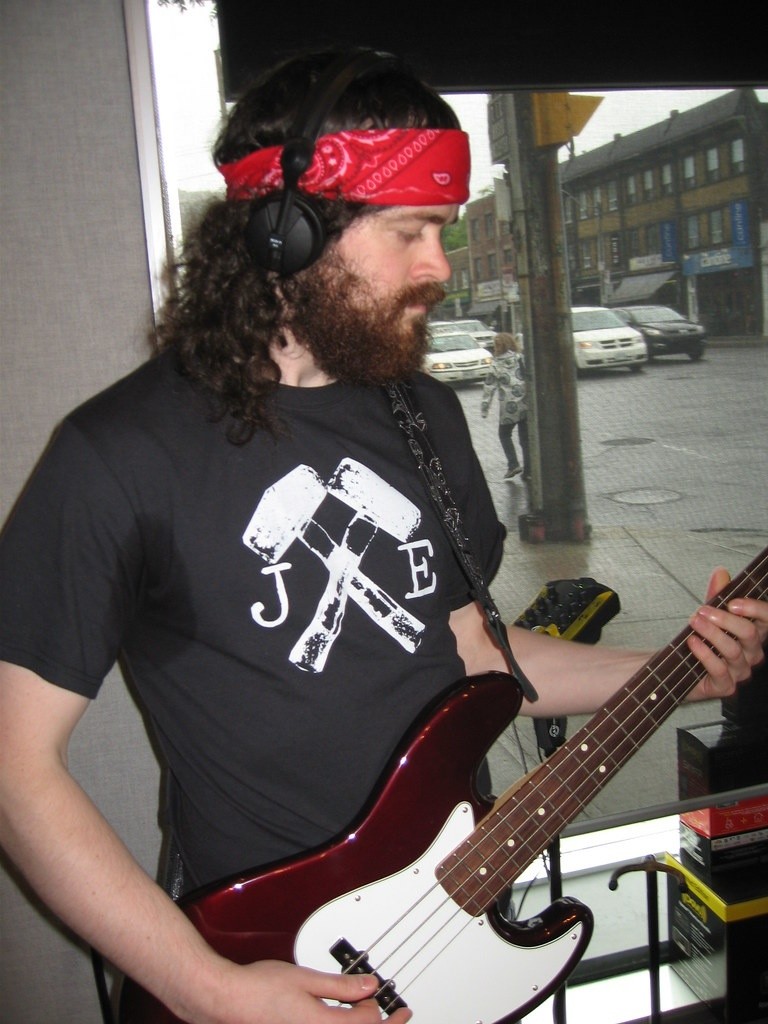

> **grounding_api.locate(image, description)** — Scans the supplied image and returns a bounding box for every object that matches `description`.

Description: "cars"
[453,319,498,350]
[612,304,705,360]
[570,306,648,378]
[423,321,493,391]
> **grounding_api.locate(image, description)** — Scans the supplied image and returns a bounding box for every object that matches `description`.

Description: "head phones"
[244,51,407,277]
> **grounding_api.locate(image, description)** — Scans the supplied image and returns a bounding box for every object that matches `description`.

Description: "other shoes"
[505,463,522,478]
[521,472,532,480]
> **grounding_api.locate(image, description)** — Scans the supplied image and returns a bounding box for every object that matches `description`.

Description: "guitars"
[116,545,767,1023]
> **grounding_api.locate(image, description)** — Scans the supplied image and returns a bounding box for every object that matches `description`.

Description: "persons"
[481,333,530,479]
[0,65,767,1024]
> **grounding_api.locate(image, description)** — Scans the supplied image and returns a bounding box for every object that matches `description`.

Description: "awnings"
[467,300,502,315]
[604,269,680,303]
